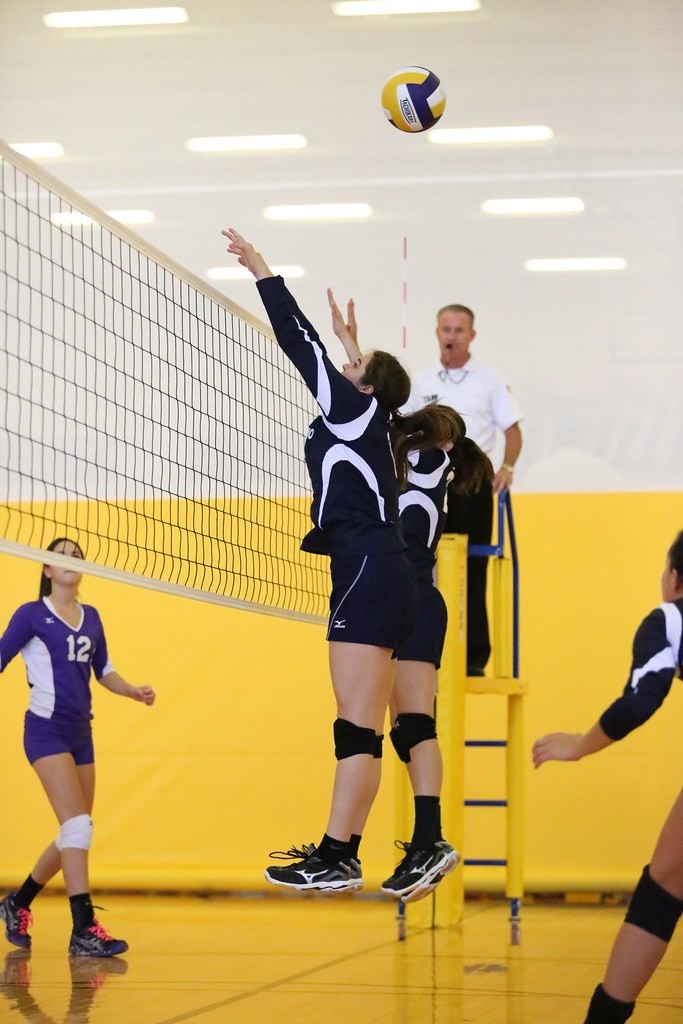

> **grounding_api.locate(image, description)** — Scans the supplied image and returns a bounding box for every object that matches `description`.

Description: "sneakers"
[381,841,460,903]
[263,843,364,893]
[68,918,128,956]
[0,892,34,948]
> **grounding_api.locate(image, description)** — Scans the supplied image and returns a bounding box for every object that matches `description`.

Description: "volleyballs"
[379,65,447,134]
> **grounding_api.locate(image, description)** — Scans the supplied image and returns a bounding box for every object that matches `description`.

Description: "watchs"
[502,464,514,473]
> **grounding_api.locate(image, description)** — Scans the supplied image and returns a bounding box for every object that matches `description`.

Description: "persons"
[398,304,525,677]
[531,529,683,1024]
[0,537,156,959]
[221,227,467,904]
